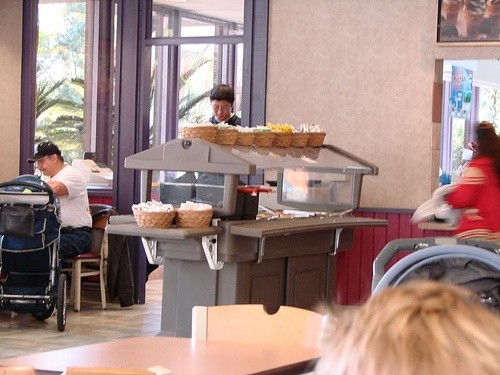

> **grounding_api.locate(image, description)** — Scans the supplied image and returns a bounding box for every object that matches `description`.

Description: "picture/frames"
[435,0,500,48]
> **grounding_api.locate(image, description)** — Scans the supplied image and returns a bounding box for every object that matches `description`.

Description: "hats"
[26,141,60,163]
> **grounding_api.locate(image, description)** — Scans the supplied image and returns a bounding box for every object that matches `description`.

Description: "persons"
[196,84,241,187]
[442,121,500,242]
[311,279,500,375]
[25,141,92,263]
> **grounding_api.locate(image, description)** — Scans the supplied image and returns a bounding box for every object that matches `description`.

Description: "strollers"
[0,174,69,331]
[370,235,500,315]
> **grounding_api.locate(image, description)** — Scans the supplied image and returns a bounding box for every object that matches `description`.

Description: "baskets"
[132,206,175,229]
[175,207,213,229]
[181,127,327,148]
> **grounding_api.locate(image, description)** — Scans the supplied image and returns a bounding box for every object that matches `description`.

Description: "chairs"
[60,202,116,313]
[191,304,337,348]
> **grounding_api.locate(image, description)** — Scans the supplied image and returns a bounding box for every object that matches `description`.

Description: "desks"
[0,333,325,375]
